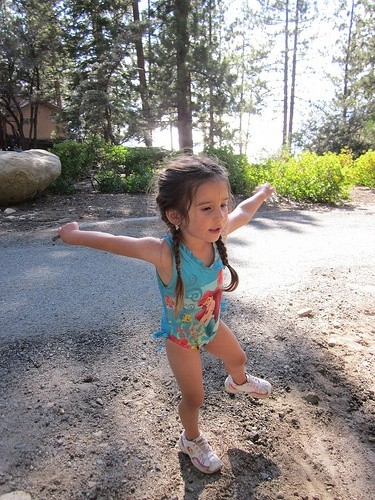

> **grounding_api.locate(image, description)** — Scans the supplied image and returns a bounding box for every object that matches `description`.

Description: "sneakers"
[224,374,272,397]
[177,431,223,474]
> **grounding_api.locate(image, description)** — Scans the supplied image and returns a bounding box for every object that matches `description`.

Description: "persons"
[57,152,277,475]
